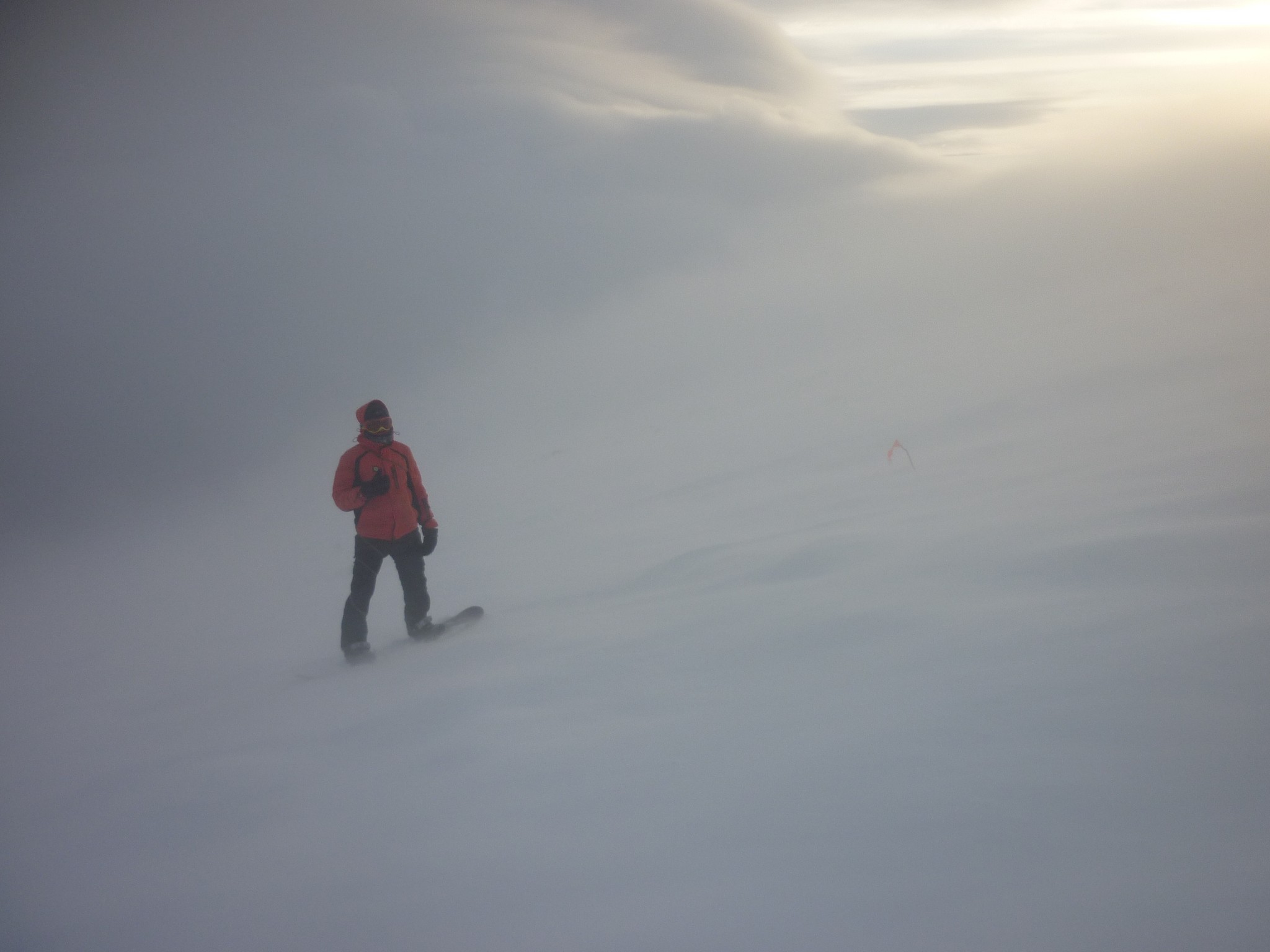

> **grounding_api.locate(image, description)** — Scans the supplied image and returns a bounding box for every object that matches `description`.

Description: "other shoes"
[407,616,445,637]
[342,632,370,651]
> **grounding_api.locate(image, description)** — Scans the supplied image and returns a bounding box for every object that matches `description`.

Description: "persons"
[332,399,444,667]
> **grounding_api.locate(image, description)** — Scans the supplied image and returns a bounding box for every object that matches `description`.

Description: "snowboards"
[402,606,485,648]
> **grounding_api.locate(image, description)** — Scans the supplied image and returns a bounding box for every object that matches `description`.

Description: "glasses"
[361,418,392,432]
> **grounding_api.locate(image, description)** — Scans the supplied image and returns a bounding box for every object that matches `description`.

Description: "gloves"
[421,528,437,555]
[362,468,390,498]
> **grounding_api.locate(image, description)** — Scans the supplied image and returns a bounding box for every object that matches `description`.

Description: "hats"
[356,400,391,423]
